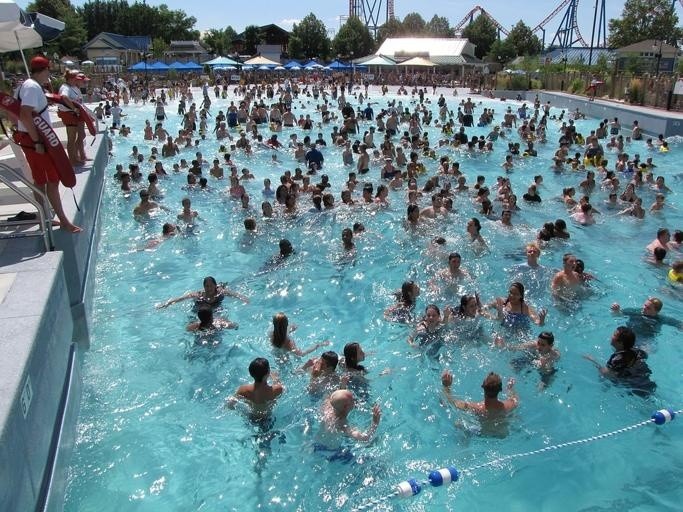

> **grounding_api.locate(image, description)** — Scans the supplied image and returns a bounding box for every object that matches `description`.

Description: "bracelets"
[33,141,42,145]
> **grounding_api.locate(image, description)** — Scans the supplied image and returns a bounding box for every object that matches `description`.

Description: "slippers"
[7,210,36,221]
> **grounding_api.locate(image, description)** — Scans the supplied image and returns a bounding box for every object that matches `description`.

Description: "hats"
[72,76,82,80]
[31,57,53,68]
[82,77,91,81]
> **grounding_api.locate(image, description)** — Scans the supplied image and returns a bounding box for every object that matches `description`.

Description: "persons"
[11,57,85,233]
[58,67,682,478]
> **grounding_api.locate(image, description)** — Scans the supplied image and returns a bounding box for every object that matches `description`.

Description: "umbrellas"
[0,2,67,78]
